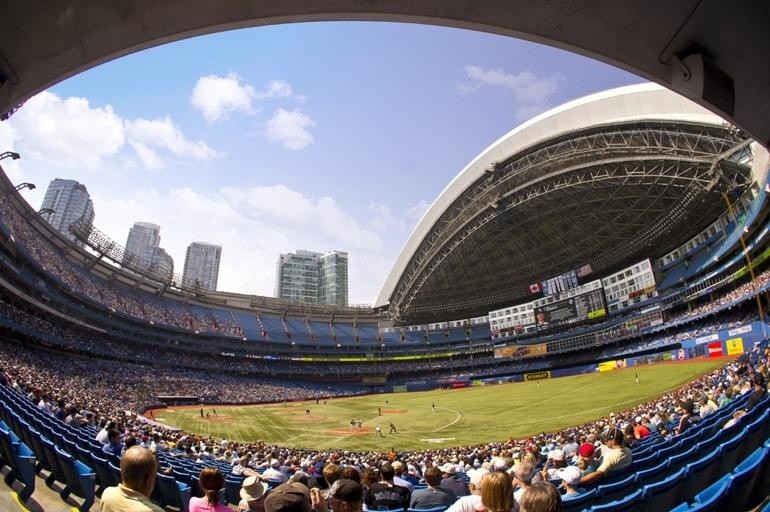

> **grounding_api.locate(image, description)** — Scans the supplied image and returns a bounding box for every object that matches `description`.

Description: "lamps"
[0,151,21,160]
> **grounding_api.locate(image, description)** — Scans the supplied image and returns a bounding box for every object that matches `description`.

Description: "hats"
[556,465,582,486]
[579,443,595,458]
[448,457,459,463]
[437,463,456,475]
[548,449,567,461]
[635,417,644,423]
[239,475,269,502]
[466,467,491,490]
[271,459,280,468]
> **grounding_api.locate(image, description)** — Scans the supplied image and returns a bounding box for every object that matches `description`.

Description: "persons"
[0,191,770,512]
